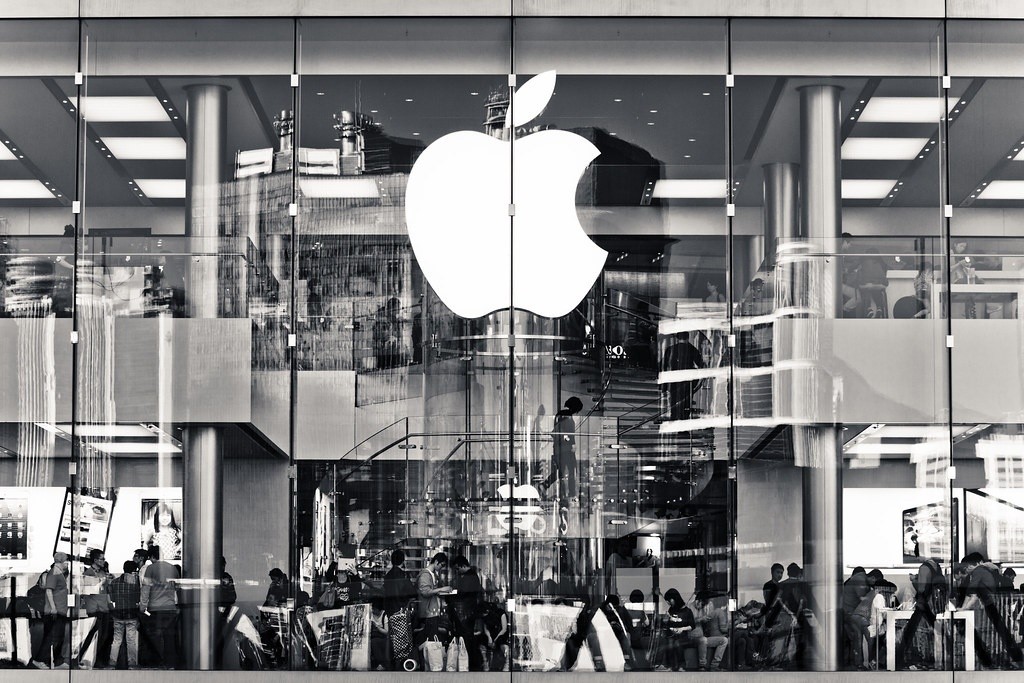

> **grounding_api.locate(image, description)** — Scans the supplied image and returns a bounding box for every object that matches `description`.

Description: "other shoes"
[375,664,386,671]
[483,661,490,672]
[752,652,767,666]
[711,666,723,672]
[103,664,116,670]
[679,667,685,672]
[92,666,104,670]
[841,660,1023,671]
[128,665,140,670]
[54,662,70,669]
[738,664,752,672]
[502,661,510,672]
[699,665,705,672]
[32,660,49,670]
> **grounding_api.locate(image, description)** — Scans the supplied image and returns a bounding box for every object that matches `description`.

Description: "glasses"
[440,561,445,568]
[96,558,105,562]
[134,555,141,558]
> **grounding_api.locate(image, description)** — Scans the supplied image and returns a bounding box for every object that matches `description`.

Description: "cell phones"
[0,498,28,573]
[53,487,120,565]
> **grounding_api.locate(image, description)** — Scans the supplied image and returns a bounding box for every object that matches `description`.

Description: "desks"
[881,610,975,671]
[928,284,1024,319]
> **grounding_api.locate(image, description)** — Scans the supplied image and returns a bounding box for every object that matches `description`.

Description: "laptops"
[964,514,988,563]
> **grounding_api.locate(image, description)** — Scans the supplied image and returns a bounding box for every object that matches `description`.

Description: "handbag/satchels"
[317,583,336,608]
[446,637,458,672]
[420,635,443,672]
[458,636,470,672]
[788,627,796,660]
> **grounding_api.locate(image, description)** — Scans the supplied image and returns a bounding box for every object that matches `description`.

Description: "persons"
[623,589,670,671]
[26,552,69,670]
[73,549,116,670]
[844,546,1024,671]
[706,231,991,320]
[686,562,812,672]
[605,594,634,670]
[140,545,181,670]
[143,504,182,560]
[343,266,378,312]
[665,588,696,671]
[101,549,151,669]
[660,333,704,435]
[221,555,237,670]
[452,555,485,672]
[383,551,417,671]
[307,278,422,371]
[636,549,656,567]
[260,567,288,620]
[625,312,661,368]
[325,550,365,610]
[540,396,583,504]
[416,552,453,641]
[555,598,568,606]
[473,601,508,672]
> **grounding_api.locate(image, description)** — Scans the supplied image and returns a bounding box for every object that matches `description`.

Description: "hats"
[53,552,67,563]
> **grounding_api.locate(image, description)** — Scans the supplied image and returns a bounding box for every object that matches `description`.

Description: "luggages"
[389,598,421,671]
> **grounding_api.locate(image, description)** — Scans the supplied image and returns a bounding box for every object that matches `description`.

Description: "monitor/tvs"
[902,498,959,564]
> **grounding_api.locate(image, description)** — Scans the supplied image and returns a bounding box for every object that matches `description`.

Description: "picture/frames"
[140,498,181,560]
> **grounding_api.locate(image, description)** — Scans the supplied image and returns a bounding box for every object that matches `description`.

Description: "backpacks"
[25,571,50,619]
[921,561,947,615]
